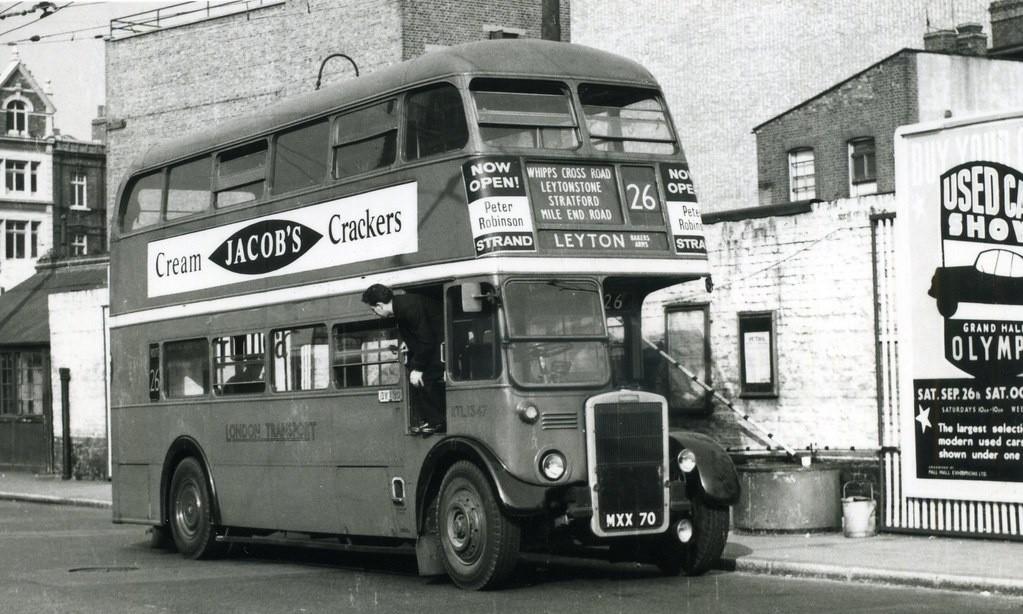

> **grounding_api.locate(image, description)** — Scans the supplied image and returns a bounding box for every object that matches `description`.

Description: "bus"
[110,28,744,593]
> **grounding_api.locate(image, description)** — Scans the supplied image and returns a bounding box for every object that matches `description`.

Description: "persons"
[569,318,605,375]
[362,284,446,434]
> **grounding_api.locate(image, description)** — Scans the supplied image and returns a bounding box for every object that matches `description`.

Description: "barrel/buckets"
[732,461,840,531]
[841,480,876,538]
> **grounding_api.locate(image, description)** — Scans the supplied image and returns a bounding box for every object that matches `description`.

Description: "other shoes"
[410,422,429,432]
[422,424,444,432]
